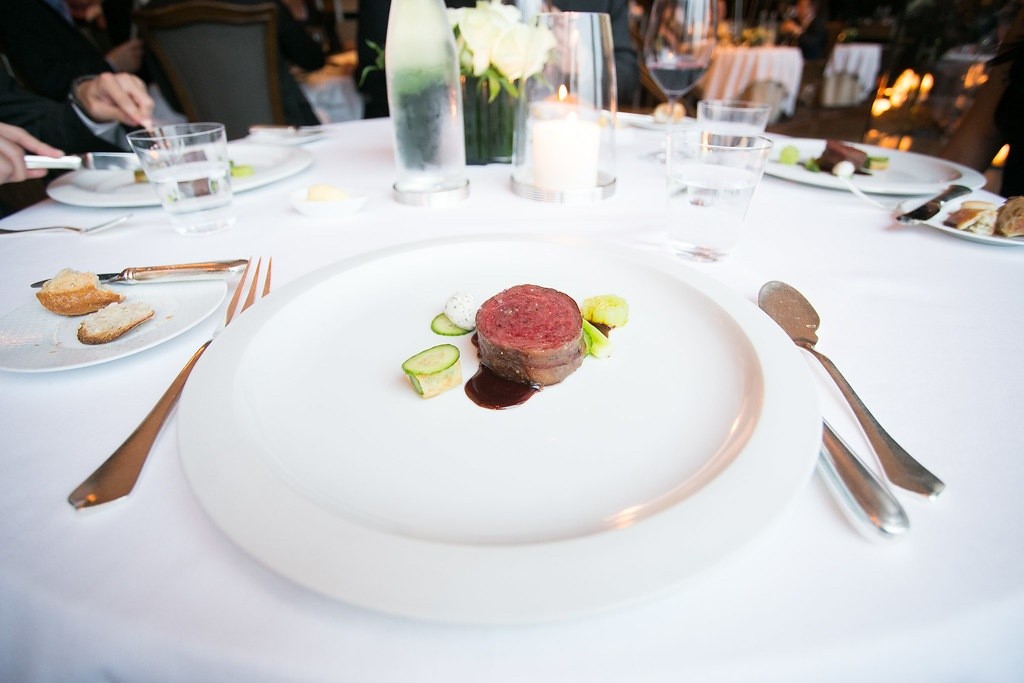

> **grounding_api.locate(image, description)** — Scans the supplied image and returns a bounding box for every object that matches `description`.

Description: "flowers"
[446,0,557,106]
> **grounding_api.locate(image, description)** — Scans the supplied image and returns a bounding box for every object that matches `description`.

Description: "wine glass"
[637,0,718,171]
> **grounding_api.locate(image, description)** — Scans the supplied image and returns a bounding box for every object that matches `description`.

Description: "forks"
[68,255,272,512]
[0,215,133,236]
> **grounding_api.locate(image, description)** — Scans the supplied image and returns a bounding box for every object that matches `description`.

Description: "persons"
[768,0,1024,200]
[348,0,725,119]
[0,0,325,220]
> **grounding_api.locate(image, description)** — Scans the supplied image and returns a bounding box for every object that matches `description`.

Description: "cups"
[696,99,772,148]
[126,122,240,234]
[665,129,773,261]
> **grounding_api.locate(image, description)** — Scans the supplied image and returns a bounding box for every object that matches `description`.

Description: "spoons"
[758,280,946,497]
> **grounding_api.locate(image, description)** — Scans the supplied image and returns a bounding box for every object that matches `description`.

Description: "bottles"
[384,0,470,206]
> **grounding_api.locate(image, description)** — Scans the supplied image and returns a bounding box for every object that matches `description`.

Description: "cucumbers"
[401,344,460,374]
[431,313,473,336]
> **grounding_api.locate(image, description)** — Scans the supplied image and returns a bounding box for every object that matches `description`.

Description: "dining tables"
[692,46,802,126]
[825,43,881,108]
[294,51,365,125]
[1,101,1024,682]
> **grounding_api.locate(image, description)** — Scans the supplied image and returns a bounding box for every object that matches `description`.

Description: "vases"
[458,71,520,167]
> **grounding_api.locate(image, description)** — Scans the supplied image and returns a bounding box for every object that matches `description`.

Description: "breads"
[34,268,125,316]
[76,301,155,344]
[942,196,1024,238]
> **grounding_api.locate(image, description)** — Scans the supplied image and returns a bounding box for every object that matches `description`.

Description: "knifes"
[895,185,973,225]
[24,153,142,171]
[31,257,248,289]
[250,126,324,132]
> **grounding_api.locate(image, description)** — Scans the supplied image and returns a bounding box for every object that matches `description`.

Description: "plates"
[247,126,331,144]
[176,232,823,626]
[764,138,987,194]
[0,248,228,373]
[629,114,697,130]
[47,142,314,207]
[898,193,1024,246]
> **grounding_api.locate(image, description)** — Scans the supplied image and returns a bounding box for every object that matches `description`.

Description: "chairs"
[135,0,284,143]
